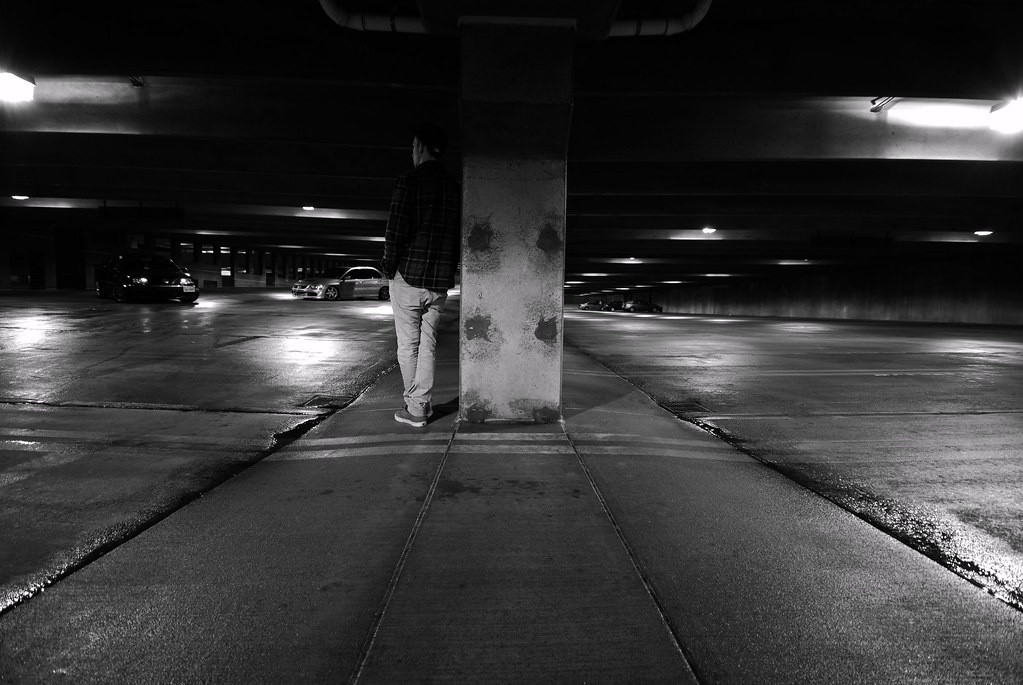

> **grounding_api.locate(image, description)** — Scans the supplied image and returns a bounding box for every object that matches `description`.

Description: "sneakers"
[393,406,435,427]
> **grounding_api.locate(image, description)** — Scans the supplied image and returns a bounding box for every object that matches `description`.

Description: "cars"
[291,266,390,301]
[580,302,604,311]
[622,300,662,313]
[604,300,624,311]
[94,249,200,303]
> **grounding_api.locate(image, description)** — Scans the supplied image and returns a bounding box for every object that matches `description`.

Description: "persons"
[381,125,462,427]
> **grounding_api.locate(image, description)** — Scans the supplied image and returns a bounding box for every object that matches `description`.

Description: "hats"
[409,121,449,159]
